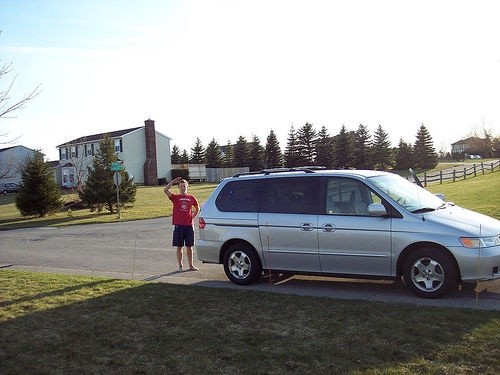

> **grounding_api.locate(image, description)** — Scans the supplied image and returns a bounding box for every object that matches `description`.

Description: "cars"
[0,182,21,195]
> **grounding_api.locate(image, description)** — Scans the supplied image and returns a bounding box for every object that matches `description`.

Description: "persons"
[163,176,201,273]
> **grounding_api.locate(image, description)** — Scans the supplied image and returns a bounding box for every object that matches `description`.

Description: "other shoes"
[179,265,183,272]
[189,266,199,271]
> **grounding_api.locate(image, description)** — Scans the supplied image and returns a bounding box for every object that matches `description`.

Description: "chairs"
[350,190,369,215]
[328,196,341,213]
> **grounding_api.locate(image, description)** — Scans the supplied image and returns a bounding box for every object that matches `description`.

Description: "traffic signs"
[111,161,125,171]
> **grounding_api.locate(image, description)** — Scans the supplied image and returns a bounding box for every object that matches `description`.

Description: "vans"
[194,165,500,299]
